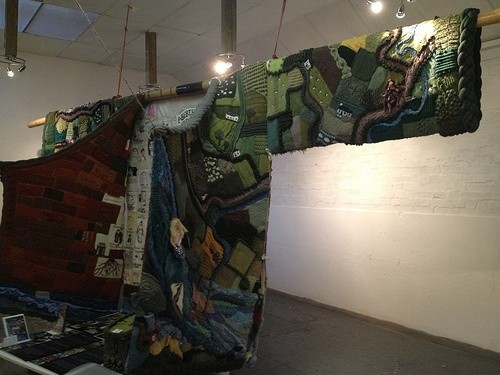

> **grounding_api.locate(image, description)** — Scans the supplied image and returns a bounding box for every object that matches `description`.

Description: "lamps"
[394,0,406,18]
[5,64,14,77]
[366,0,383,13]
[241,57,246,68]
[212,54,233,75]
